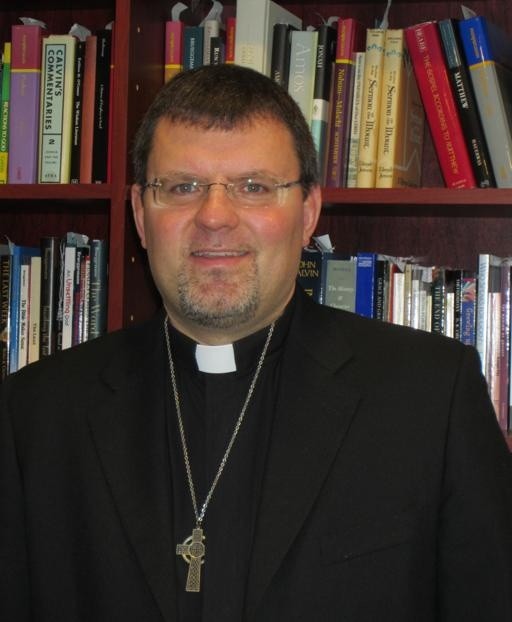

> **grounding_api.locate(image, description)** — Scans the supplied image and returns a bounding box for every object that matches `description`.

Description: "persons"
[0,63,512,621]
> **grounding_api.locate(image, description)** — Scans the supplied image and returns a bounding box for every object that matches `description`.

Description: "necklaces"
[164,314,278,593]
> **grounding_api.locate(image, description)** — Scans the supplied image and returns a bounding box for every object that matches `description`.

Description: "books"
[164,0,512,189]
[0,25,109,185]
[0,232,109,378]
[295,251,511,430]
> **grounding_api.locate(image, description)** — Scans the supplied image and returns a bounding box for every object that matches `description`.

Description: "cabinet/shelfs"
[1,35,512,455]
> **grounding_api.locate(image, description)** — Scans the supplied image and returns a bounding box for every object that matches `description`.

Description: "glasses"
[142,177,303,207]
